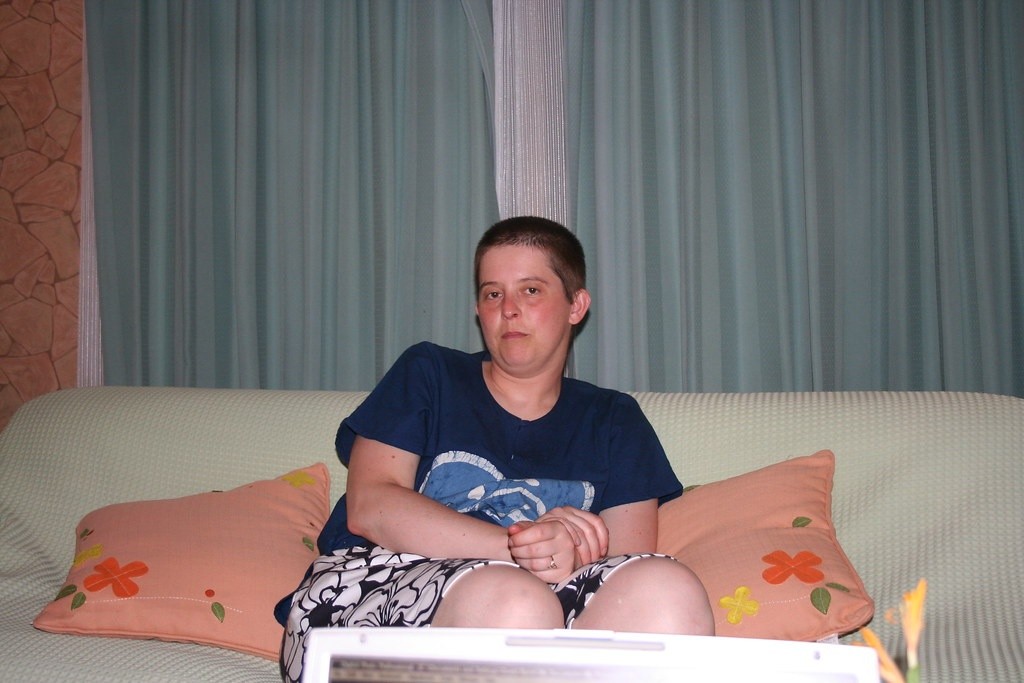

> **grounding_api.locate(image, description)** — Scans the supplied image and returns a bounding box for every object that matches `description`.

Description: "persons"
[281,216,714,682]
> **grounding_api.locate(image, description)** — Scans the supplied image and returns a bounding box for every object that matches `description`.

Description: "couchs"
[0,385,1024,683]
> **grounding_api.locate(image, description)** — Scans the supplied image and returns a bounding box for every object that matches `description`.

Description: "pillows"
[656,451,876,641]
[31,462,330,663]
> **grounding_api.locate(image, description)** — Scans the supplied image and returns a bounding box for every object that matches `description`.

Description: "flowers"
[850,577,932,683]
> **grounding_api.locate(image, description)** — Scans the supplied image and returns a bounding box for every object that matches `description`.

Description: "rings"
[551,556,558,569]
